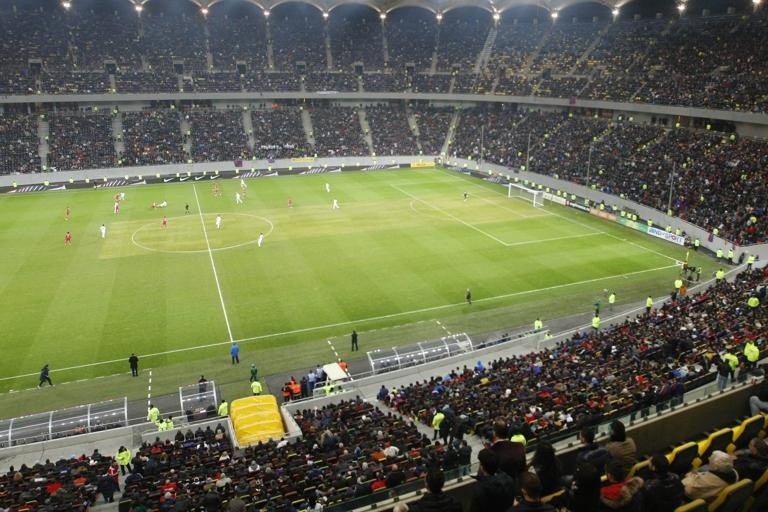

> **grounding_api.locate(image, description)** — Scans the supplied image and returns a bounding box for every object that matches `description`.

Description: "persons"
[63,179,339,248]
[120,353,767,511]
[38,364,53,388]
[446,16,768,268]
[535,318,542,329]
[0,418,132,510]
[466,289,472,304]
[1,1,489,173]
[230,343,240,364]
[351,331,359,352]
[550,260,768,357]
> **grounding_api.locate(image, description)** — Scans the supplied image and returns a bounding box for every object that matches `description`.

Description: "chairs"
[1,286,767,512]
